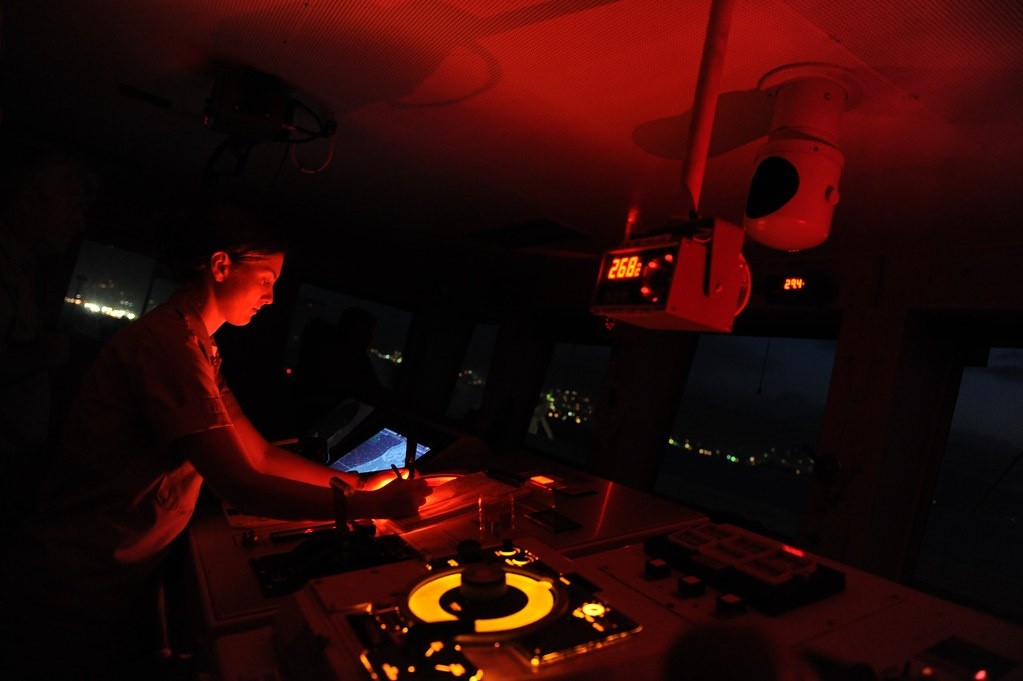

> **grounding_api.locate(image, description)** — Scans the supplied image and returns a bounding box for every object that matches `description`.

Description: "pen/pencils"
[390,463,404,480]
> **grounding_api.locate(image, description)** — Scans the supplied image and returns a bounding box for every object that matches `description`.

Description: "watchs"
[345,470,366,493]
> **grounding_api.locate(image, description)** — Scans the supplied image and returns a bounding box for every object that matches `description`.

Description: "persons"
[2,207,435,681]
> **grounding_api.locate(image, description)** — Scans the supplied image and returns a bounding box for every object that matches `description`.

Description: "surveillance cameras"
[740,138,846,255]
